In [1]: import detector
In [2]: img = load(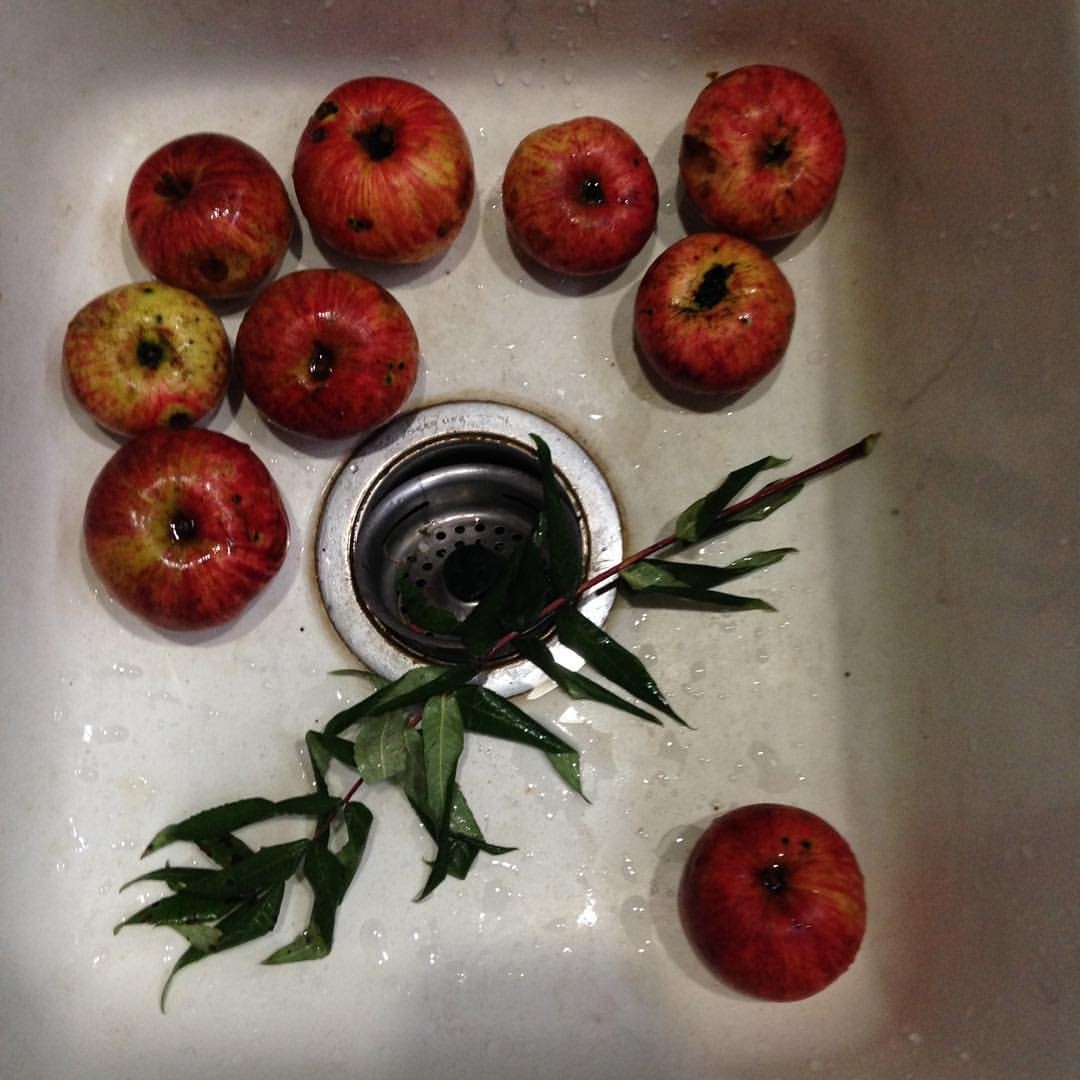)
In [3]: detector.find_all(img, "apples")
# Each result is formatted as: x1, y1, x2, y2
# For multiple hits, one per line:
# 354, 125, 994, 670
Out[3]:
82, 428, 289, 634
124, 133, 293, 306
502, 115, 660, 277
61, 281, 231, 438
680, 63, 847, 242
636, 231, 797, 395
293, 75, 475, 263
234, 268, 418, 441
677, 803, 868, 1002
